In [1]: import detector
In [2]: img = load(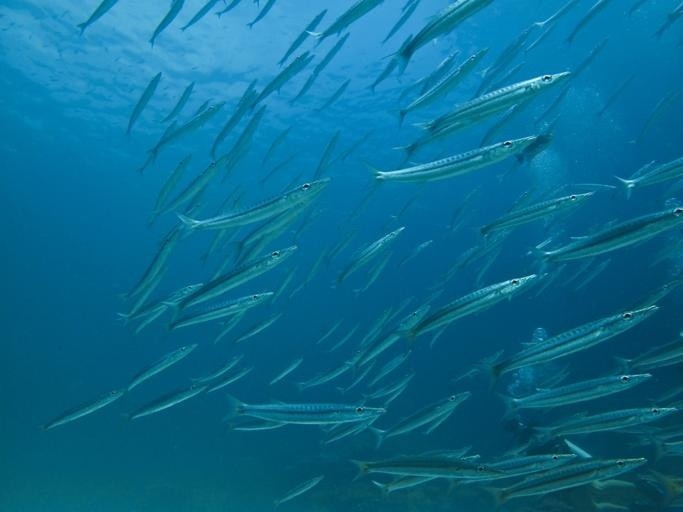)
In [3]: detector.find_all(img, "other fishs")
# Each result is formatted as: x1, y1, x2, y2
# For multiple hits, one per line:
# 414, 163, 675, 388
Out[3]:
46, 0, 682, 510
